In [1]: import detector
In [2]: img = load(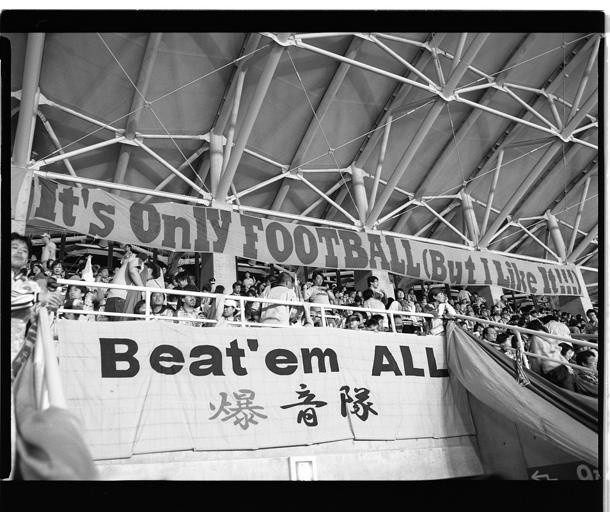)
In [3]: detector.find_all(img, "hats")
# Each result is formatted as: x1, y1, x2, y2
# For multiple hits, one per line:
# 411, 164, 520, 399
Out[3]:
40, 233, 51, 239
